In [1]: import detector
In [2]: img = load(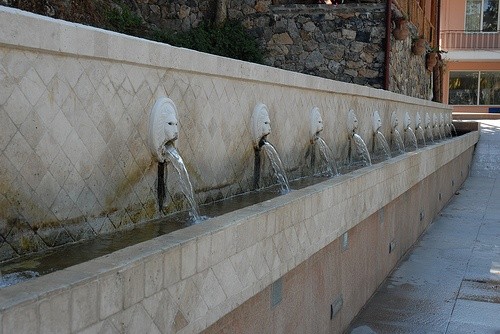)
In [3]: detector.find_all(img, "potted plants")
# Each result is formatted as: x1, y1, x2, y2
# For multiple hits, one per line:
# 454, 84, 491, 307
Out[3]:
411, 32, 425, 55
425, 48, 437, 70
392, 9, 408, 40
